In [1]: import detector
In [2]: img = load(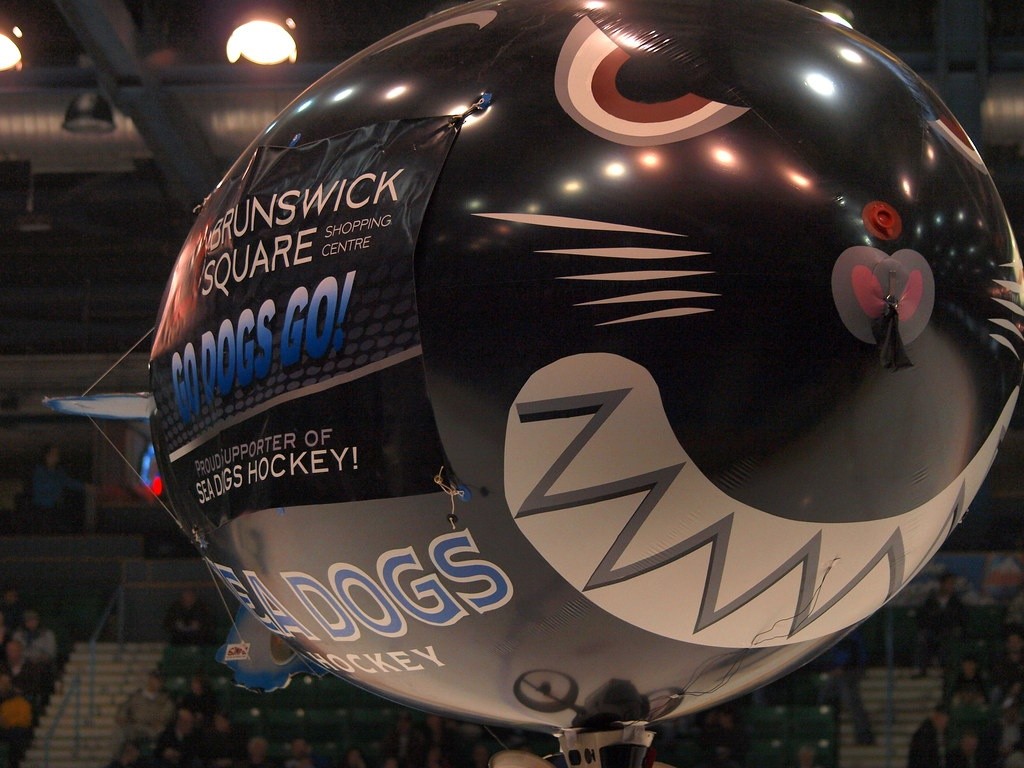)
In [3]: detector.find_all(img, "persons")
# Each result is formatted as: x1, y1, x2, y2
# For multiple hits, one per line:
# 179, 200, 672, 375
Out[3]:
0, 443, 1024, 768
126, 669, 174, 746
